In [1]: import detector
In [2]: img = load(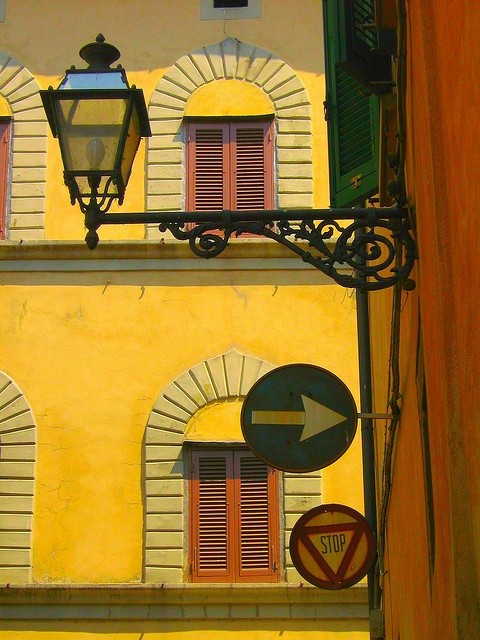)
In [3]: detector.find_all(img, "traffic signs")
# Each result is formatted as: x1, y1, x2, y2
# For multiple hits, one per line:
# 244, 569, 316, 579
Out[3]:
288, 502, 373, 590
